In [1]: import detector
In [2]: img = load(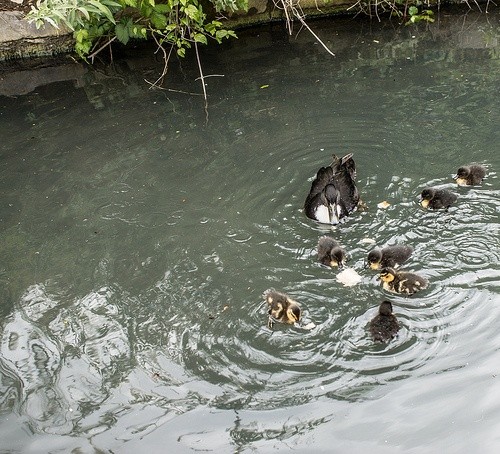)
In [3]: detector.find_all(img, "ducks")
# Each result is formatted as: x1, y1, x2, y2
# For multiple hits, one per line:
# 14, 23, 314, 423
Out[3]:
366, 244, 413, 271
264, 290, 302, 327
317, 236, 347, 269
452, 162, 486, 187
420, 188, 457, 211
304, 151, 359, 225
375, 266, 428, 297
369, 299, 400, 345
